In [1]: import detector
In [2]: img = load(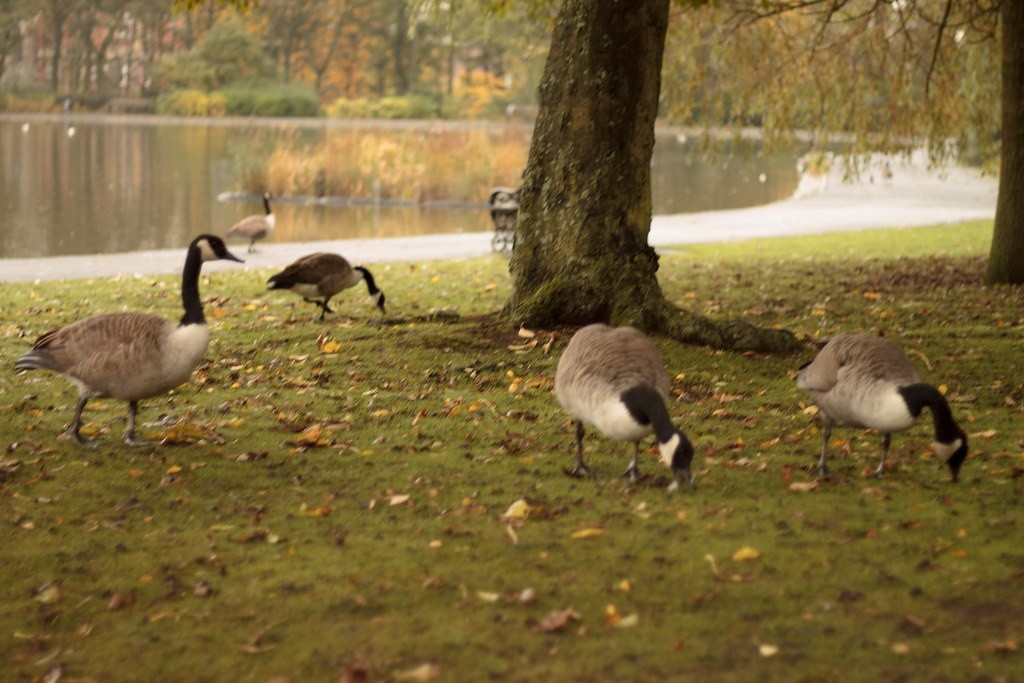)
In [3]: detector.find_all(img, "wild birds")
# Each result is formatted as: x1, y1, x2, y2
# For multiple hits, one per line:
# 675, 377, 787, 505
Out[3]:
266, 253, 387, 323
553, 324, 695, 495
795, 334, 969, 483
225, 191, 275, 253
11, 232, 247, 446
